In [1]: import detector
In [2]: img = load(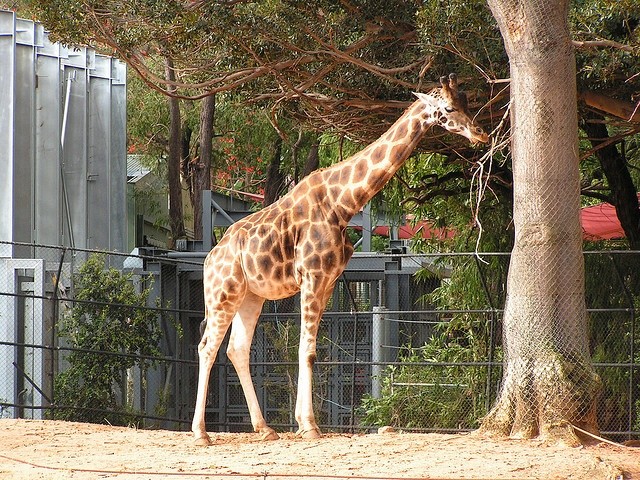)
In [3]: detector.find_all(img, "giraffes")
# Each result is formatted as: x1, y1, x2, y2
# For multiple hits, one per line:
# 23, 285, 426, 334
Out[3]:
192, 74, 489, 447
